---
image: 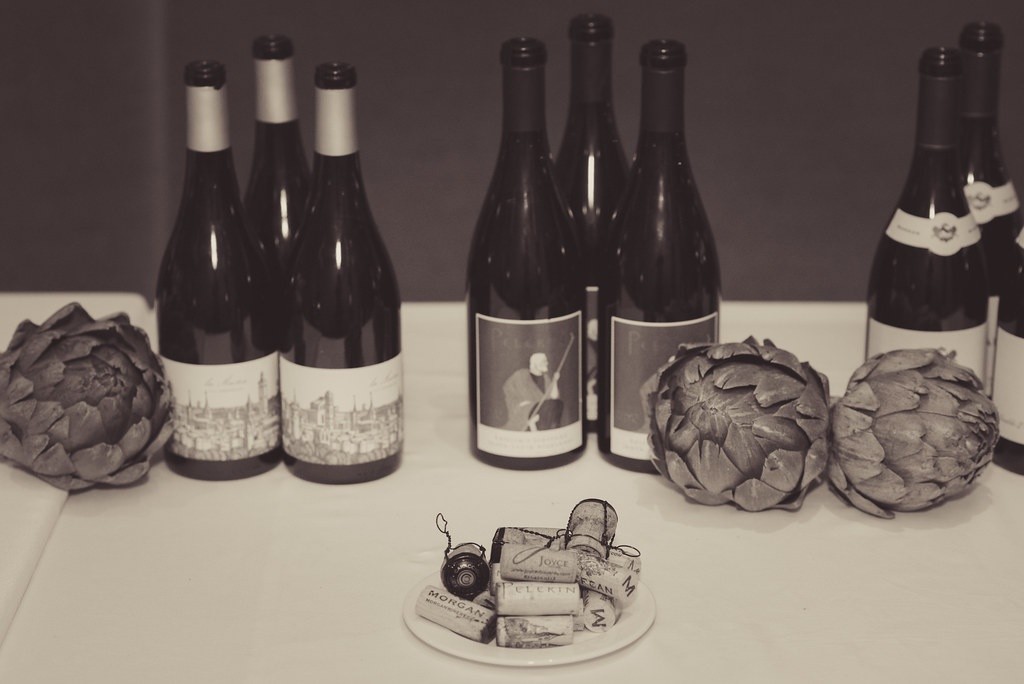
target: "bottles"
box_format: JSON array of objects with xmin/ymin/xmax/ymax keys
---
[
  {"xmin": 553, "ymin": 14, "xmax": 627, "ymax": 432},
  {"xmin": 468, "ymin": 37, "xmax": 586, "ymax": 470},
  {"xmin": 157, "ymin": 59, "xmax": 282, "ymax": 480},
  {"xmin": 279, "ymin": 62, "xmax": 404, "ymax": 484},
  {"xmin": 244, "ymin": 35, "xmax": 310, "ymax": 305},
  {"xmin": 866, "ymin": 45, "xmax": 989, "ymax": 389},
  {"xmin": 959, "ymin": 20, "xmax": 1021, "ymax": 397},
  {"xmin": 598, "ymin": 38, "xmax": 720, "ymax": 474},
  {"xmin": 993, "ymin": 228, "xmax": 1024, "ymax": 475}
]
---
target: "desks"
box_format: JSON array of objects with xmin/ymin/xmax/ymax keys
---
[{"xmin": 0, "ymin": 293, "xmax": 1024, "ymax": 684}]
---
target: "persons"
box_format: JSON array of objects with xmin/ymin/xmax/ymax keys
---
[{"xmin": 519, "ymin": 351, "xmax": 565, "ymax": 431}]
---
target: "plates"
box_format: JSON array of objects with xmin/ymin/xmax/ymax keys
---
[{"xmin": 403, "ymin": 569, "xmax": 655, "ymax": 667}]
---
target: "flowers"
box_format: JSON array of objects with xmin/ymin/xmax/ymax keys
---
[
  {"xmin": 825, "ymin": 350, "xmax": 1001, "ymax": 519},
  {"xmin": 639, "ymin": 337, "xmax": 831, "ymax": 512},
  {"xmin": 0, "ymin": 302, "xmax": 174, "ymax": 489}
]
